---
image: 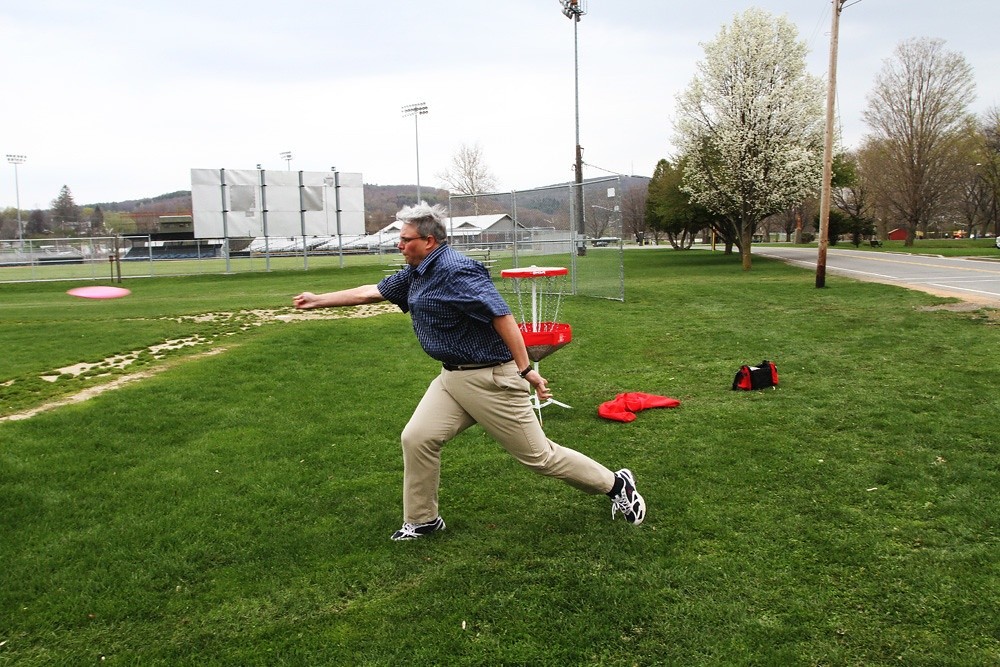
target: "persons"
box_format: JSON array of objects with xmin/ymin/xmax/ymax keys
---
[{"xmin": 294, "ymin": 204, "xmax": 648, "ymax": 541}]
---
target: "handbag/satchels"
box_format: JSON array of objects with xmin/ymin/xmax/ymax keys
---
[{"xmin": 732, "ymin": 360, "xmax": 779, "ymax": 390}]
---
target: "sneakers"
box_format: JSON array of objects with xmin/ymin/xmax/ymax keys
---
[
  {"xmin": 390, "ymin": 516, "xmax": 446, "ymax": 541},
  {"xmin": 611, "ymin": 467, "xmax": 646, "ymax": 525}
]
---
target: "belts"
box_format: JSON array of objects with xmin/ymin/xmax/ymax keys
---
[{"xmin": 443, "ymin": 358, "xmax": 513, "ymax": 372}]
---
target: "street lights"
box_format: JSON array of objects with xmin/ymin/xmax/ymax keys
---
[
  {"xmin": 555, "ymin": 0, "xmax": 589, "ymax": 258},
  {"xmin": 399, "ymin": 101, "xmax": 429, "ymax": 207},
  {"xmin": 279, "ymin": 150, "xmax": 292, "ymax": 172},
  {"xmin": 5, "ymin": 154, "xmax": 27, "ymax": 249}
]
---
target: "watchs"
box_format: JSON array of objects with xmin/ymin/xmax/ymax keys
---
[{"xmin": 517, "ymin": 364, "xmax": 532, "ymax": 378}]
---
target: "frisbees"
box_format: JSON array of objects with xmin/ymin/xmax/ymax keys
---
[{"xmin": 67, "ymin": 286, "xmax": 131, "ymax": 299}]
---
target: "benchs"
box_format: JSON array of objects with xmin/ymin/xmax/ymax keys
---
[
  {"xmin": 465, "ymin": 250, "xmax": 490, "ymax": 261},
  {"xmin": 385, "ymin": 266, "xmax": 492, "ymax": 279},
  {"xmin": 870, "ymin": 240, "xmax": 883, "ymax": 248}
]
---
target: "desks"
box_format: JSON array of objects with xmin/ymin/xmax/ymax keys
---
[{"xmin": 389, "ymin": 259, "xmax": 497, "ymax": 265}]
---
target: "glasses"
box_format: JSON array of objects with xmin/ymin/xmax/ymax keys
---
[{"xmin": 399, "ymin": 235, "xmax": 428, "ymax": 243}]
